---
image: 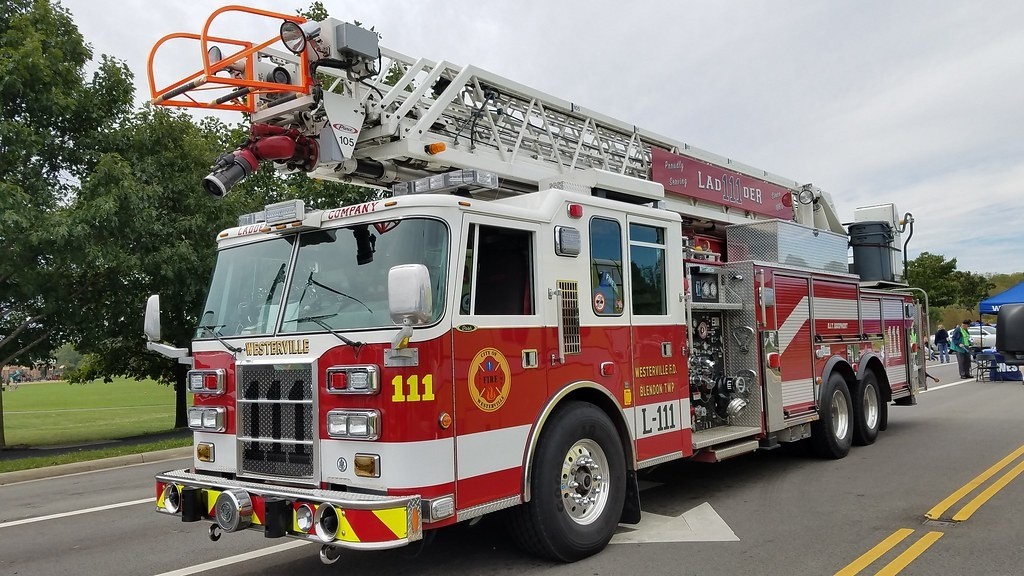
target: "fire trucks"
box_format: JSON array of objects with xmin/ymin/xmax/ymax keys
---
[{"xmin": 144, "ymin": 5, "xmax": 939, "ymax": 560}]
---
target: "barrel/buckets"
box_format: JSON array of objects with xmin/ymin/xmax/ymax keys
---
[
  {"xmin": 850, "ymin": 233, "xmax": 893, "ymax": 282},
  {"xmin": 849, "ymin": 221, "xmax": 890, "ymax": 235}
]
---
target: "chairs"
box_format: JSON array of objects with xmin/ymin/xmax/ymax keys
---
[{"xmin": 968, "ymin": 347, "xmax": 1004, "ymax": 383}]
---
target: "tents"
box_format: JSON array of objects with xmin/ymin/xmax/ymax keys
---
[{"xmin": 978, "ymin": 281, "xmax": 1024, "ymax": 377}]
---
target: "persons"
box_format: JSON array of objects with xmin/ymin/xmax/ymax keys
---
[
  {"xmin": 950, "ymin": 321, "xmax": 974, "ymax": 379},
  {"xmin": 934, "ymin": 325, "xmax": 950, "ymax": 363},
  {"xmin": 923, "ymin": 337, "xmax": 938, "ymax": 362}
]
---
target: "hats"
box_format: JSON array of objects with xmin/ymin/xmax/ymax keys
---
[{"xmin": 962, "ymin": 320, "xmax": 969, "ymax": 324}]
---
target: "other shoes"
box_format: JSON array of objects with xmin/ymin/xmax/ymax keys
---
[
  {"xmin": 961, "ymin": 375, "xmax": 966, "ymax": 379},
  {"xmin": 966, "ymin": 374, "xmax": 974, "ymax": 378}
]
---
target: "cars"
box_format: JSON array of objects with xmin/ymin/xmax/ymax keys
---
[
  {"xmin": 928, "ymin": 327, "xmax": 996, "ymax": 350},
  {"xmin": 975, "ymin": 324, "xmax": 996, "ymax": 334}
]
